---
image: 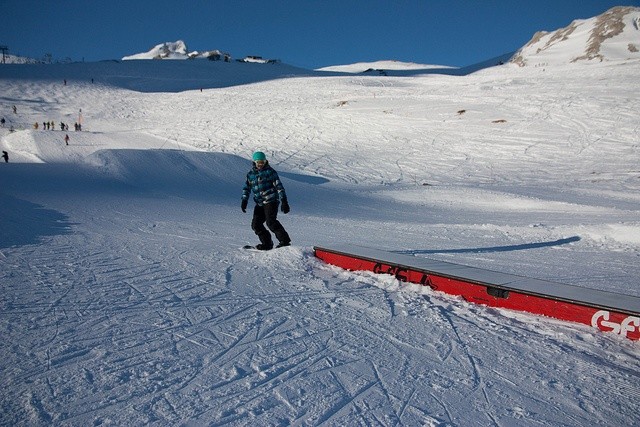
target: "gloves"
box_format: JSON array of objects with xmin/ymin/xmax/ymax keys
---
[
  {"xmin": 281, "ymin": 197, "xmax": 290, "ymax": 213},
  {"xmin": 241, "ymin": 199, "xmax": 248, "ymax": 213}
]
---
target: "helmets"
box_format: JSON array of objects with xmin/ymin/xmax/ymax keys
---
[{"xmin": 252, "ymin": 151, "xmax": 266, "ymax": 162}]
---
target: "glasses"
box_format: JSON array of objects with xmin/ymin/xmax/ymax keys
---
[{"xmin": 255, "ymin": 160, "xmax": 266, "ymax": 165}]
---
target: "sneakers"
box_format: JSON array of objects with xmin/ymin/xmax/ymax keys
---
[
  {"xmin": 256, "ymin": 243, "xmax": 273, "ymax": 250},
  {"xmin": 277, "ymin": 241, "xmax": 290, "ymax": 248}
]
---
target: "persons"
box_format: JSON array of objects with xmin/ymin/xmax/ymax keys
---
[
  {"xmin": 59, "ymin": 122, "xmax": 66, "ymax": 131},
  {"xmin": 74, "ymin": 122, "xmax": 82, "ymax": 131},
  {"xmin": 2, "ymin": 150, "xmax": 9, "ymax": 163},
  {"xmin": 33, "ymin": 121, "xmax": 38, "ymax": 128},
  {"xmin": 43, "ymin": 122, "xmax": 46, "ymax": 130},
  {"xmin": 241, "ymin": 151, "xmax": 291, "ymax": 250},
  {"xmin": 51, "ymin": 121, "xmax": 55, "ymax": 130},
  {"xmin": 64, "ymin": 134, "xmax": 69, "ymax": 145},
  {"xmin": 66, "ymin": 124, "xmax": 69, "ymax": 131},
  {"xmin": 46, "ymin": 121, "xmax": 51, "ymax": 130}
]
